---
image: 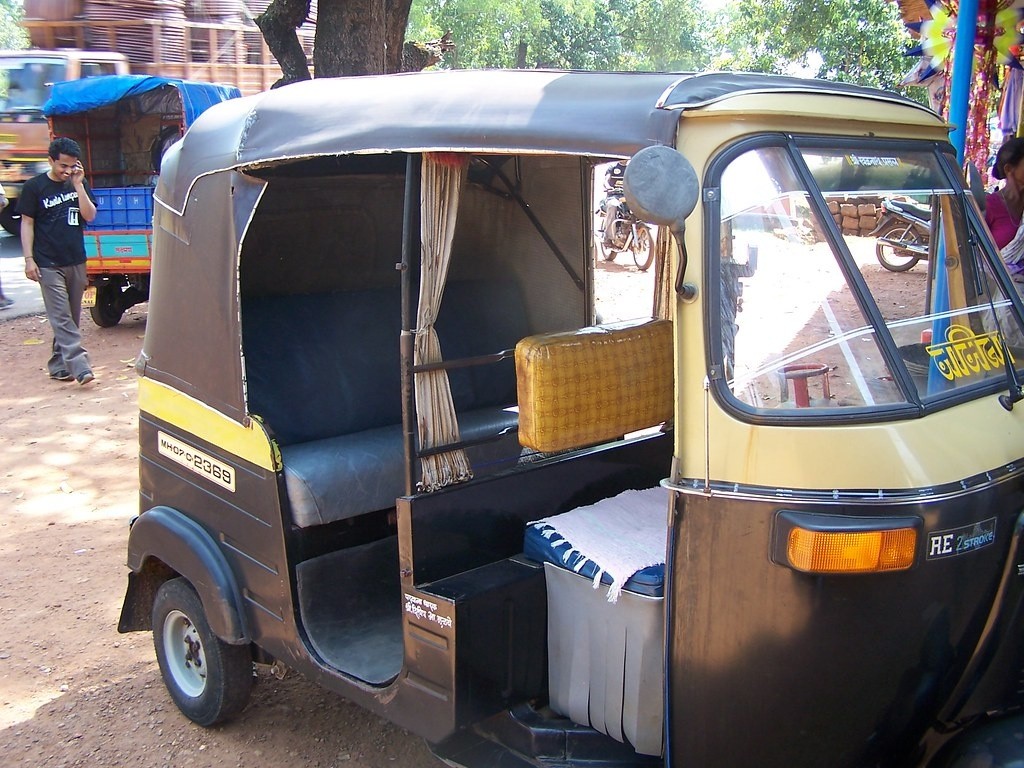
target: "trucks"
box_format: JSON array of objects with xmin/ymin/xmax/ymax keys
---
[{"xmin": 0, "ymin": 20, "xmax": 315, "ymax": 237}]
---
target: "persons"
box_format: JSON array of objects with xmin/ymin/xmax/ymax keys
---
[
  {"xmin": 0, "ymin": 182, "xmax": 16, "ymax": 310},
  {"xmin": 980, "ymin": 137, "xmax": 1024, "ymax": 348},
  {"xmin": 14, "ymin": 138, "xmax": 100, "ymax": 386},
  {"xmin": 603, "ymin": 160, "xmax": 628, "ymax": 246}
]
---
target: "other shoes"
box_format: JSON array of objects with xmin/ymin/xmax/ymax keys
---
[{"xmin": 604, "ymin": 237, "xmax": 612, "ymax": 244}]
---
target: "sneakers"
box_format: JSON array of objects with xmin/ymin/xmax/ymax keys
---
[
  {"xmin": 78, "ymin": 370, "xmax": 95, "ymax": 384},
  {"xmin": 0, "ymin": 297, "xmax": 16, "ymax": 310},
  {"xmin": 50, "ymin": 370, "xmax": 75, "ymax": 382}
]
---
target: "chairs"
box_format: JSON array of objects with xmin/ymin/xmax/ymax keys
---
[{"xmin": 516, "ymin": 314, "xmax": 674, "ymax": 755}]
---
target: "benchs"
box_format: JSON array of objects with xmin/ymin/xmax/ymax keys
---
[{"xmin": 244, "ymin": 273, "xmax": 530, "ymax": 562}]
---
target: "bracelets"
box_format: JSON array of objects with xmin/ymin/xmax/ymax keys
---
[{"xmin": 25, "ymin": 256, "xmax": 34, "ymax": 259}]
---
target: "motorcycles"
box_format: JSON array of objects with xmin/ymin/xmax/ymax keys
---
[
  {"xmin": 595, "ymin": 176, "xmax": 656, "ymax": 273},
  {"xmin": 867, "ymin": 194, "xmax": 932, "ymax": 273},
  {"xmin": 115, "ymin": 63, "xmax": 1022, "ymax": 767},
  {"xmin": 45, "ymin": 81, "xmax": 187, "ymax": 329}
]
